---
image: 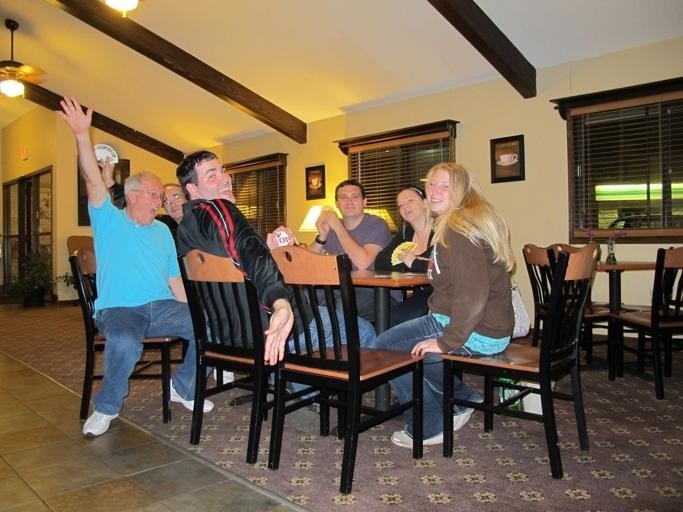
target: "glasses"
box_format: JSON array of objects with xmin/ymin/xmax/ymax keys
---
[{"xmin": 129, "ymin": 190, "xmax": 165, "ymax": 199}]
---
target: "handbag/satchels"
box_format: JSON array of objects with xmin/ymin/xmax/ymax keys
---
[{"xmin": 510, "ymin": 287, "xmax": 530, "ymax": 339}]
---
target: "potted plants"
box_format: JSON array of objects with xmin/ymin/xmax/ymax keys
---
[{"xmin": 5, "ymin": 242, "xmax": 77, "ymax": 307}]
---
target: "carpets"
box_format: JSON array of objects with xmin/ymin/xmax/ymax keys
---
[{"xmin": 0, "ymin": 308, "xmax": 681, "ymax": 512}]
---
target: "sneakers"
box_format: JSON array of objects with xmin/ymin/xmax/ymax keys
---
[
  {"xmin": 82, "ymin": 410, "xmax": 119, "ymax": 437},
  {"xmin": 391, "ymin": 430, "xmax": 443, "ymax": 448},
  {"xmin": 453, "ymin": 400, "xmax": 484, "ymax": 432},
  {"xmin": 170, "ymin": 378, "xmax": 215, "ymax": 412},
  {"xmin": 213, "ymin": 369, "xmax": 234, "ymax": 385}
]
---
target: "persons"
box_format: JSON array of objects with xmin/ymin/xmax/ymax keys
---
[
  {"xmin": 371, "ymin": 186, "xmax": 444, "ymax": 327},
  {"xmin": 96, "ymin": 158, "xmax": 189, "ymax": 234},
  {"xmin": 368, "ymin": 163, "xmax": 515, "ymax": 449},
  {"xmin": 175, "ymin": 150, "xmax": 376, "ymax": 400},
  {"xmin": 304, "ymin": 180, "xmax": 402, "ymax": 323},
  {"xmin": 55, "ymin": 96, "xmax": 214, "ymax": 439}
]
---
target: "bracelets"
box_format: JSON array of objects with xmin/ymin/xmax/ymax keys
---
[{"xmin": 315, "ymin": 235, "xmax": 327, "ymax": 245}]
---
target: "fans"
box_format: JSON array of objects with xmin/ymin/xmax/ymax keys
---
[{"xmin": 0, "ymin": 19, "xmax": 44, "ymax": 84}]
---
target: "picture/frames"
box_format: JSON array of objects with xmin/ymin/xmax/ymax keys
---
[
  {"xmin": 490, "ymin": 135, "xmax": 524, "ymax": 184},
  {"xmin": 305, "ymin": 165, "xmax": 325, "ymax": 201}
]
